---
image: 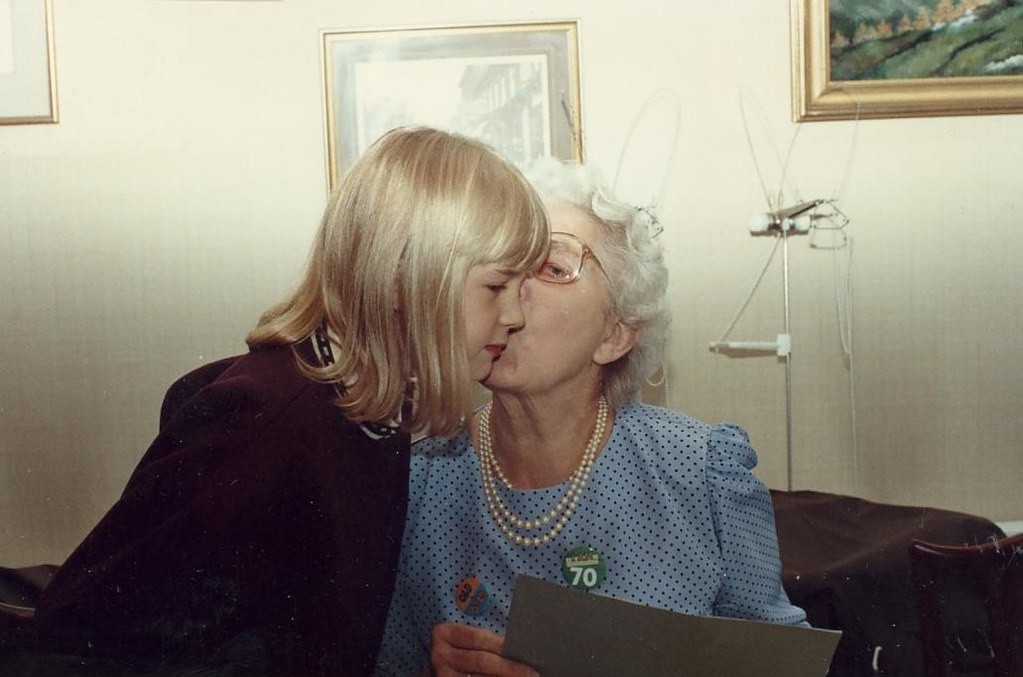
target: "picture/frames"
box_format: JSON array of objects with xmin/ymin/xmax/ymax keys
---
[
  {"xmin": 317, "ymin": 16, "xmax": 587, "ymax": 202},
  {"xmin": 787, "ymin": 0, "xmax": 1023, "ymax": 123},
  {"xmin": 0, "ymin": 0, "xmax": 58, "ymax": 125}
]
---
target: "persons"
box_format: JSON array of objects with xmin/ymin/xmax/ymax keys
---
[
  {"xmin": 376, "ymin": 159, "xmax": 811, "ymax": 677},
  {"xmin": 0, "ymin": 124, "xmax": 552, "ymax": 677}
]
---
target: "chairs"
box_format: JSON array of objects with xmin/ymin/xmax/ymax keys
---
[{"xmin": 906, "ymin": 533, "xmax": 1023, "ymax": 676}]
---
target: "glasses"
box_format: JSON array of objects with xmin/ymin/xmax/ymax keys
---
[{"xmin": 532, "ymin": 231, "xmax": 617, "ymax": 308}]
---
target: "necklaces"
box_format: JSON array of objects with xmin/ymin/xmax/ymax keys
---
[{"xmin": 479, "ymin": 395, "xmax": 607, "ymax": 545}]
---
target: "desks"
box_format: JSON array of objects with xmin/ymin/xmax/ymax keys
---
[{"xmin": 767, "ymin": 489, "xmax": 1023, "ymax": 677}]
